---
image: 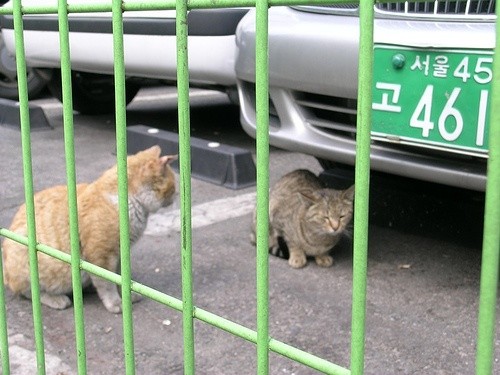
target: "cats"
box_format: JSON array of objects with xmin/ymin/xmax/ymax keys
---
[
  {"xmin": 250, "ymin": 168, "xmax": 355, "ymax": 269},
  {"xmin": 2, "ymin": 144, "xmax": 180, "ymax": 314}
]
